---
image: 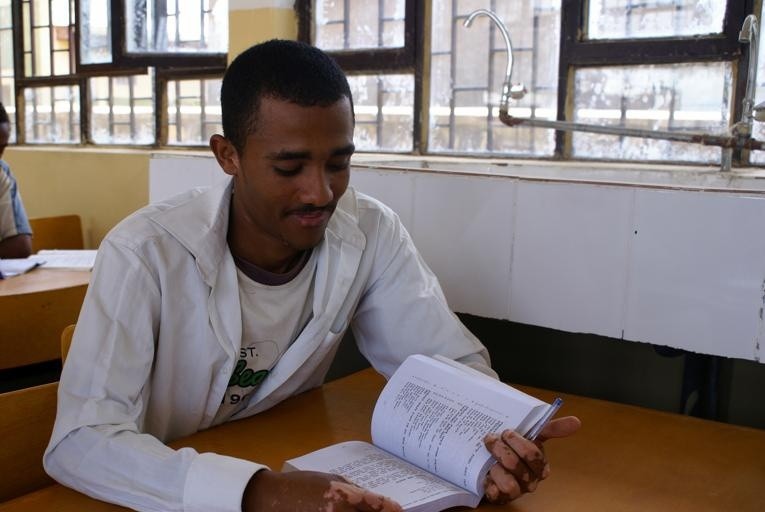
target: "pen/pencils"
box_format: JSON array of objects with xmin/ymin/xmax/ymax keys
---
[{"xmin": 525, "ymin": 397, "xmax": 564, "ymax": 442}]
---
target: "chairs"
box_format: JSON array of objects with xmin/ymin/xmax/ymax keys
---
[{"xmin": 29, "ymin": 215, "xmax": 87, "ymax": 256}]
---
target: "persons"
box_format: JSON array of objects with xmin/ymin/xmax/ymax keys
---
[
  {"xmin": 0, "ymin": 101, "xmax": 34, "ymax": 258},
  {"xmin": 43, "ymin": 39, "xmax": 582, "ymax": 512}
]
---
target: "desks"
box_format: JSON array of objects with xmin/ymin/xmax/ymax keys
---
[{"xmin": 1, "ymin": 258, "xmax": 765, "ymax": 511}]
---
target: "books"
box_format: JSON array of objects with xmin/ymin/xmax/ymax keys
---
[
  {"xmin": 0, "ymin": 259, "xmax": 48, "ymax": 279},
  {"xmin": 281, "ymin": 353, "xmax": 554, "ymax": 512},
  {"xmin": 28, "ymin": 249, "xmax": 99, "ymax": 272}
]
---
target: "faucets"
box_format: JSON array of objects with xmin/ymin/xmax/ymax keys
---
[
  {"xmin": 738, "ymin": 14, "xmax": 765, "ymax": 128},
  {"xmin": 463, "ymin": 8, "xmax": 526, "ymax": 115}
]
